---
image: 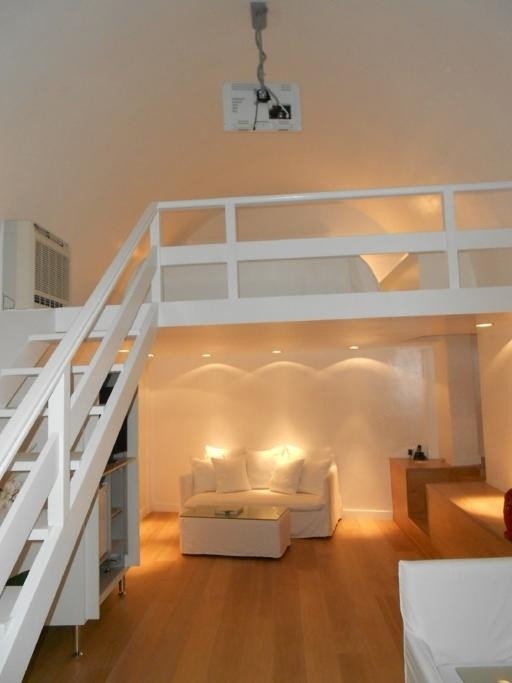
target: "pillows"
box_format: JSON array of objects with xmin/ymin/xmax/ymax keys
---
[{"xmin": 185, "ymin": 443, "xmax": 335, "ymax": 496}]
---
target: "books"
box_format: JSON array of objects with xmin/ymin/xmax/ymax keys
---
[{"xmin": 215, "ymin": 506, "xmax": 242, "ymax": 515}]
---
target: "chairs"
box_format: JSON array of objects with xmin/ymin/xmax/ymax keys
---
[{"xmin": 395, "ymin": 557, "xmax": 512, "ymax": 683}]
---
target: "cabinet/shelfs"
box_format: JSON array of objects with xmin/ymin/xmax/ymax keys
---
[{"xmin": 32, "ymin": 364, "xmax": 142, "ymax": 658}]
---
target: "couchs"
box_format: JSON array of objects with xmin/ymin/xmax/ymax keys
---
[{"xmin": 178, "ymin": 446, "xmax": 344, "ymax": 539}]
